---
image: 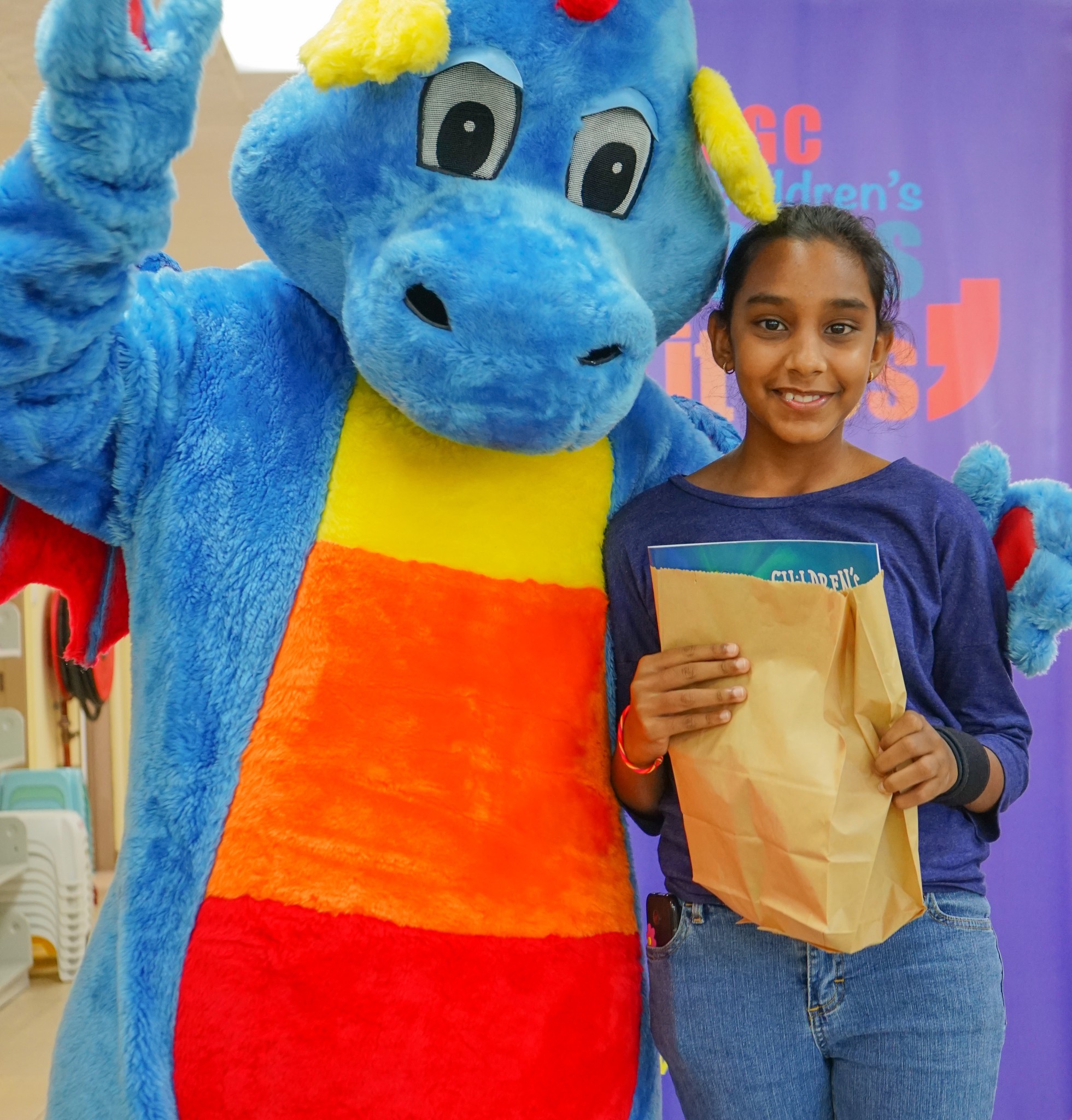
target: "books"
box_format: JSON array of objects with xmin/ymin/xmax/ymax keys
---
[{"xmin": 648, "ymin": 539, "xmax": 883, "ymax": 594}]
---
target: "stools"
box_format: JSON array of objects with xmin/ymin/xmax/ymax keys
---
[{"xmin": 0, "ymin": 765, "xmax": 96, "ymax": 983}]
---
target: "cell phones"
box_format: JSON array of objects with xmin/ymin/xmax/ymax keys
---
[{"xmin": 646, "ymin": 892, "xmax": 681, "ymax": 947}]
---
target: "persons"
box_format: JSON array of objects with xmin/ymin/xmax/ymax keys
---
[{"xmin": 595, "ymin": 201, "xmax": 1037, "ymax": 1120}]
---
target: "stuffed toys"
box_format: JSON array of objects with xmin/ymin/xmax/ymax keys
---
[{"xmin": 0, "ymin": 0, "xmax": 1072, "ymax": 1120}]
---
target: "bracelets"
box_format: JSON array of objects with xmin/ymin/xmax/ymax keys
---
[
  {"xmin": 618, "ymin": 704, "xmax": 663, "ymax": 774},
  {"xmin": 926, "ymin": 725, "xmax": 992, "ymax": 807}
]
